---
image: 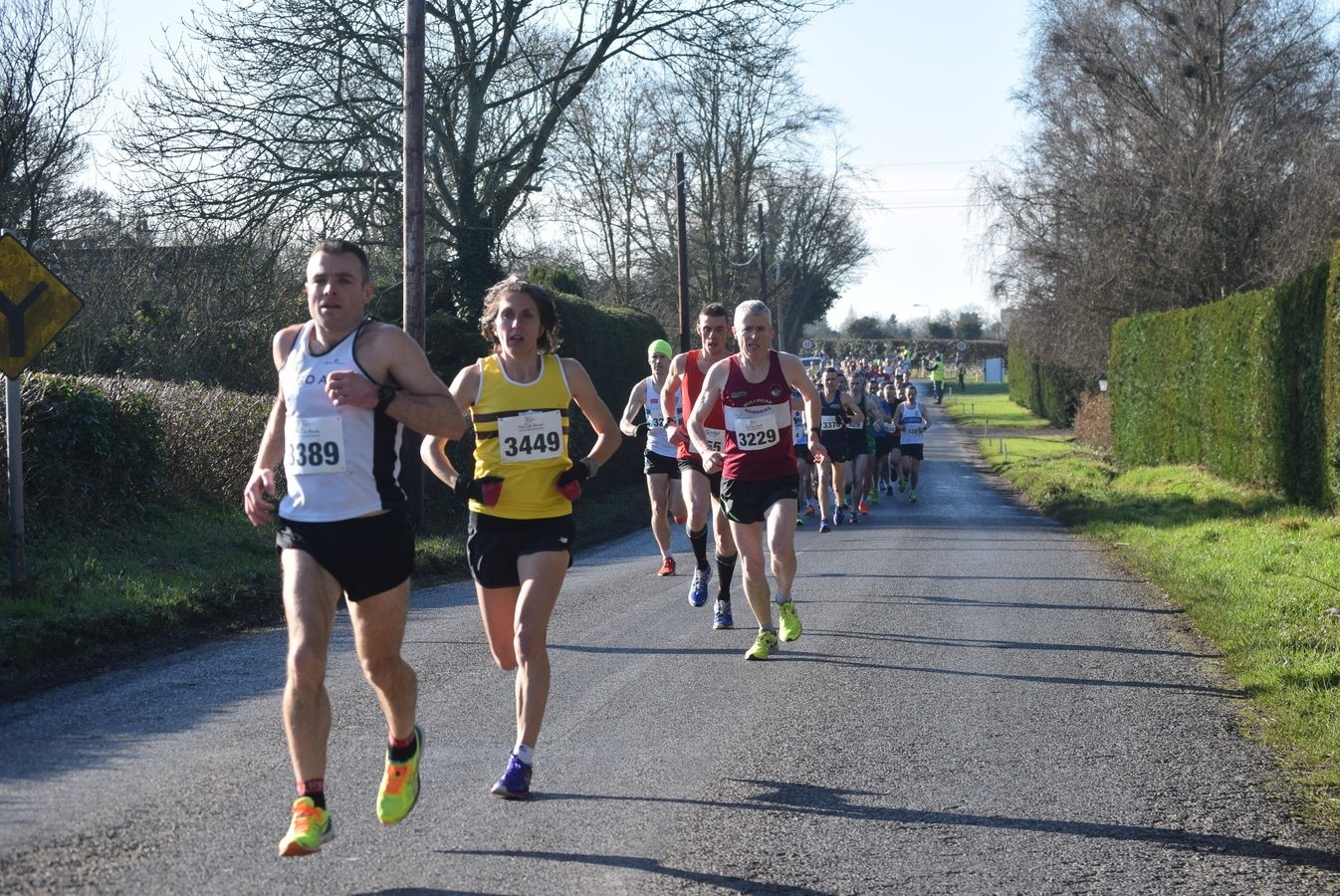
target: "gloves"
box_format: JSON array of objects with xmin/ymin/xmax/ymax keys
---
[
  {"xmin": 635, "ymin": 422, "xmax": 651, "ymax": 437},
  {"xmin": 453, "ymin": 474, "xmax": 504, "ymax": 507},
  {"xmin": 554, "ymin": 461, "xmax": 590, "ymax": 501}
]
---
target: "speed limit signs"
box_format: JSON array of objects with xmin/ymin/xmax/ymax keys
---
[
  {"xmin": 957, "ymin": 341, "xmax": 968, "ymax": 352},
  {"xmin": 802, "ymin": 340, "xmax": 812, "ymax": 351}
]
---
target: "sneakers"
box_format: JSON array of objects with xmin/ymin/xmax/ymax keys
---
[
  {"xmin": 279, "ymin": 796, "xmax": 336, "ymax": 855},
  {"xmin": 745, "ymin": 629, "xmax": 779, "ymax": 660},
  {"xmin": 775, "ymin": 595, "xmax": 803, "ymax": 642},
  {"xmin": 848, "ymin": 510, "xmax": 860, "ymax": 524},
  {"xmin": 812, "ymin": 468, "xmax": 917, "ymax": 503},
  {"xmin": 491, "ymin": 750, "xmax": 532, "ymax": 797},
  {"xmin": 805, "ymin": 505, "xmax": 814, "ymax": 515},
  {"xmin": 819, "ymin": 518, "xmax": 830, "ymax": 533},
  {"xmin": 688, "ymin": 564, "xmax": 713, "ymax": 607},
  {"xmin": 713, "ymin": 598, "xmax": 733, "ymax": 629},
  {"xmin": 674, "ymin": 515, "xmax": 686, "ymax": 524},
  {"xmin": 376, "ymin": 725, "xmax": 425, "ymax": 825},
  {"xmin": 796, "ymin": 517, "xmax": 804, "ymax": 526},
  {"xmin": 658, "ymin": 556, "xmax": 676, "ymax": 576},
  {"xmin": 859, "ymin": 501, "xmax": 868, "ymax": 515},
  {"xmin": 833, "ymin": 503, "xmax": 845, "ymax": 526}
]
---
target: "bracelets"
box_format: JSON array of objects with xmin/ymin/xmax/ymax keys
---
[
  {"xmin": 579, "ymin": 456, "xmax": 597, "ymax": 477},
  {"xmin": 923, "ymin": 426, "xmax": 926, "ymax": 430}
]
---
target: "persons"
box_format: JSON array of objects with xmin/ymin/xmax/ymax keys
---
[
  {"xmin": 686, "ymin": 299, "xmax": 829, "ymax": 661},
  {"xmin": 618, "ymin": 339, "xmax": 688, "ymax": 579},
  {"xmin": 790, "ymin": 347, "xmax": 944, "ymax": 534},
  {"xmin": 419, "ymin": 274, "xmax": 623, "ymax": 802},
  {"xmin": 660, "ymin": 303, "xmax": 738, "ymax": 629},
  {"xmin": 956, "ymin": 357, "xmax": 967, "ymax": 392},
  {"xmin": 244, "ymin": 238, "xmax": 465, "ymax": 857}
]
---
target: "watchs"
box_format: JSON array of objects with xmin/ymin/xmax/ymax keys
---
[
  {"xmin": 662, "ymin": 417, "xmax": 676, "ymax": 429},
  {"xmin": 811, "ymin": 427, "xmax": 821, "ymax": 438},
  {"xmin": 372, "ymin": 383, "xmax": 395, "ymax": 414}
]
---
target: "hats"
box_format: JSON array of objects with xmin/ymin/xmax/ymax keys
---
[{"xmin": 648, "ymin": 339, "xmax": 672, "ymax": 361}]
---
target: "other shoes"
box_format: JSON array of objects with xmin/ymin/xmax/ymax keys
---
[{"xmin": 844, "ymin": 502, "xmax": 849, "ymax": 509}]
